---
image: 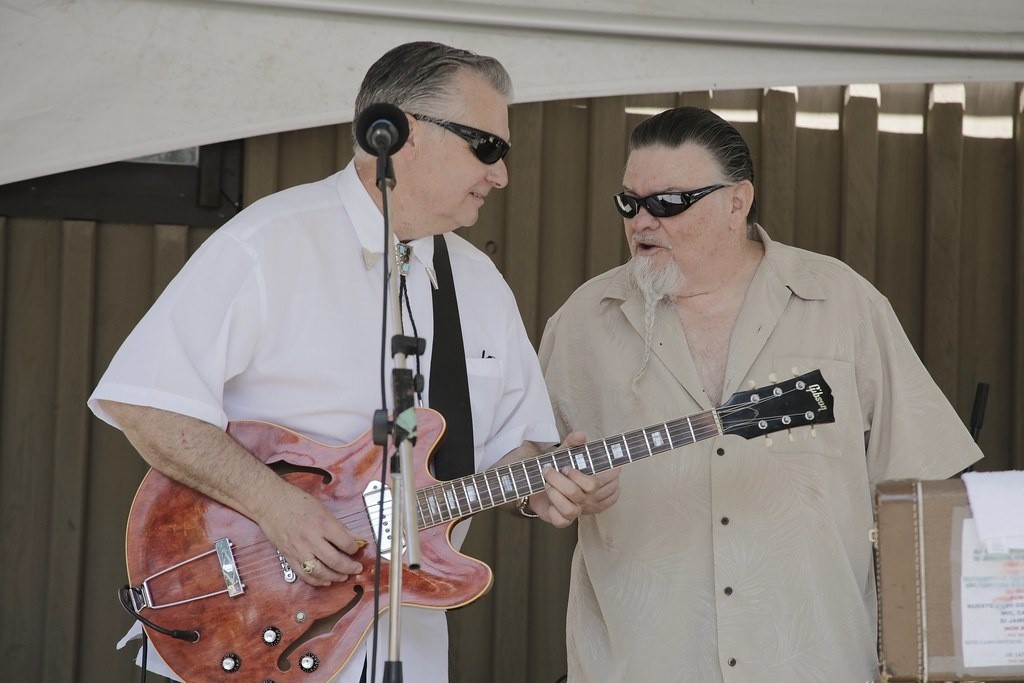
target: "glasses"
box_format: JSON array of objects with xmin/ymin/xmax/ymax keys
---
[
  {"xmin": 614, "ymin": 183, "xmax": 731, "ymax": 219},
  {"xmin": 406, "ymin": 112, "xmax": 510, "ymax": 164}
]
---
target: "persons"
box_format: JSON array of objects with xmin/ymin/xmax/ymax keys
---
[
  {"xmin": 535, "ymin": 106, "xmax": 986, "ymax": 683},
  {"xmin": 89, "ymin": 41, "xmax": 595, "ymax": 683}
]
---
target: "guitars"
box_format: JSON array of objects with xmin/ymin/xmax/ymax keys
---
[{"xmin": 119, "ymin": 358, "xmax": 839, "ymax": 682}]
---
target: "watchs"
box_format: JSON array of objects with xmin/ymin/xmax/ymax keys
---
[{"xmin": 516, "ymin": 497, "xmax": 539, "ymax": 518}]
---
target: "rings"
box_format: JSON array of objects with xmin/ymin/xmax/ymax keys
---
[{"xmin": 301, "ymin": 559, "xmax": 316, "ymax": 572}]
